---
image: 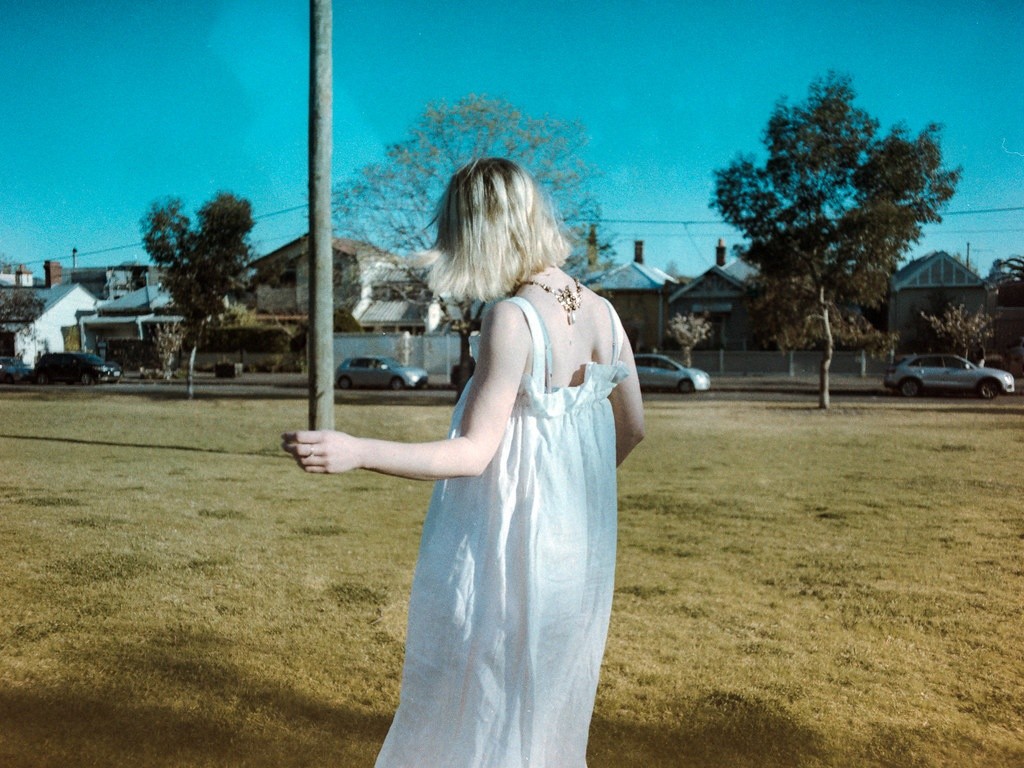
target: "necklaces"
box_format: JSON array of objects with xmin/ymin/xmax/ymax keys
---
[{"xmin": 512, "ymin": 277, "xmax": 583, "ymax": 324}]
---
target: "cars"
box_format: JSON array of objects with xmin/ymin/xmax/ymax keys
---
[{"xmin": 633, "ymin": 354, "xmax": 711, "ymax": 393}]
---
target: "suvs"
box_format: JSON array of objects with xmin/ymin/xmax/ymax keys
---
[
  {"xmin": 0, "ymin": 357, "xmax": 35, "ymax": 385},
  {"xmin": 334, "ymin": 354, "xmax": 429, "ymax": 391},
  {"xmin": 33, "ymin": 351, "xmax": 123, "ymax": 385},
  {"xmin": 882, "ymin": 352, "xmax": 1016, "ymax": 400}
]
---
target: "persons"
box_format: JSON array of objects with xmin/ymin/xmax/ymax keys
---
[{"xmin": 282, "ymin": 156, "xmax": 648, "ymax": 768}]
